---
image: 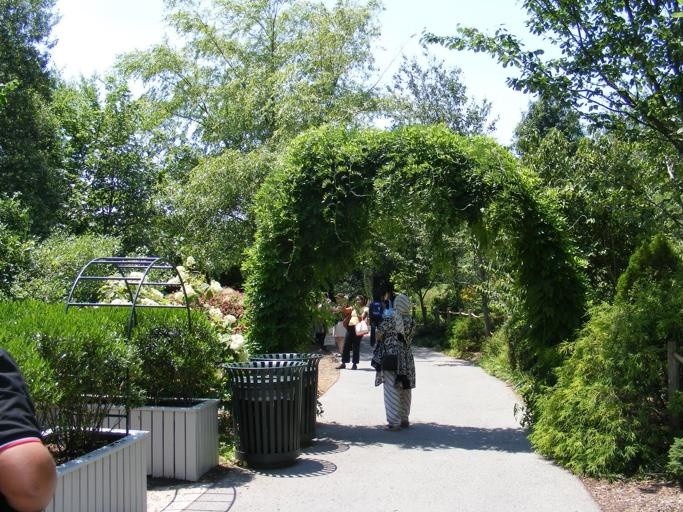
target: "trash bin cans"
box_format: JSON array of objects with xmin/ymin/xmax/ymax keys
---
[
  {"xmin": 248, "ymin": 350, "xmax": 325, "ymax": 446},
  {"xmin": 221, "ymin": 359, "xmax": 310, "ymax": 469}
]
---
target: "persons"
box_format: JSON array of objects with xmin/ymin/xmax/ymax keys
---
[
  {"xmin": 314, "ymin": 297, "xmax": 331, "ymax": 354},
  {"xmin": 333, "ymin": 294, "xmax": 371, "ymax": 370},
  {"xmin": 328, "ymin": 292, "xmax": 349, "ymax": 357},
  {"xmin": 0, "ymin": 347, "xmax": 58, "ymax": 511},
  {"xmin": 368, "ymin": 289, "xmax": 384, "ymax": 347},
  {"xmin": 371, "ymin": 294, "xmax": 415, "ymax": 431}
]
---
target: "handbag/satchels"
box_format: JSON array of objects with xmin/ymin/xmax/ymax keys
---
[{"xmin": 355, "ymin": 320, "xmax": 368, "ymax": 336}]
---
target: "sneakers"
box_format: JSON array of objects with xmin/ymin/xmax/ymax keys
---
[
  {"xmin": 350, "ymin": 365, "xmax": 357, "ymax": 369},
  {"xmin": 383, "ymin": 422, "xmax": 409, "ymax": 432},
  {"xmin": 335, "ymin": 363, "xmax": 346, "ymax": 369}
]
